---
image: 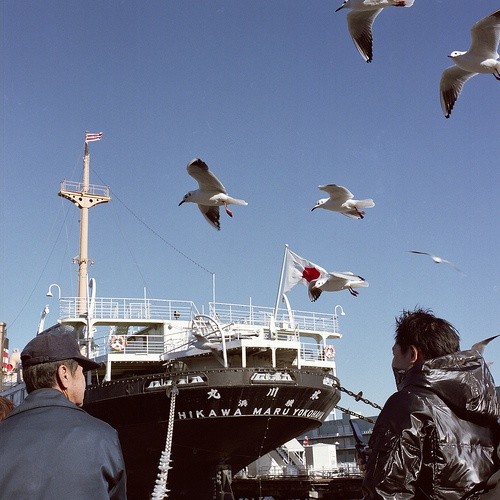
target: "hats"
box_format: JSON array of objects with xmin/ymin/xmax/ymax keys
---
[{"xmin": 20, "ymin": 331, "xmax": 100, "ymax": 372}]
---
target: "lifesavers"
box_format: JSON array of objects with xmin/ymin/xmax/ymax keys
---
[
  {"xmin": 324, "ymin": 346, "xmax": 336, "ymax": 358},
  {"xmin": 110, "ymin": 336, "xmax": 126, "ymax": 350}
]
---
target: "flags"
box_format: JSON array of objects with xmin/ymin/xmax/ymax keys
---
[
  {"xmin": 2, "ymin": 337, "xmax": 10, "ymax": 372},
  {"xmin": 86, "ymin": 132, "xmax": 103, "ymax": 142},
  {"xmin": 284, "ymin": 250, "xmax": 328, "ymax": 295}
]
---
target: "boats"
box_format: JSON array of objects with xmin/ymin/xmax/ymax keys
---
[{"xmin": 0, "ymin": 131, "xmax": 346, "ymax": 477}]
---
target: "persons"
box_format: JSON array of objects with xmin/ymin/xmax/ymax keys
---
[
  {"xmin": 354, "ymin": 305, "xmax": 500, "ymax": 500},
  {"xmin": 0, "ymin": 332, "xmax": 128, "ymax": 500}
]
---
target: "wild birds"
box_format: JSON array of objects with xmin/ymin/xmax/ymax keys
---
[
  {"xmin": 407, "ymin": 251, "xmax": 461, "ymax": 273},
  {"xmin": 309, "ymin": 272, "xmax": 369, "ymax": 302},
  {"xmin": 440, "ymin": 9, "xmax": 500, "ymax": 119},
  {"xmin": 336, "ymin": 0, "xmax": 415, "ymax": 63},
  {"xmin": 311, "ymin": 183, "xmax": 375, "ymax": 220},
  {"xmin": 179, "ymin": 158, "xmax": 249, "ymax": 231},
  {"xmin": 173, "ymin": 311, "xmax": 180, "ymax": 320},
  {"xmin": 470, "ymin": 334, "xmax": 500, "ymax": 366}
]
